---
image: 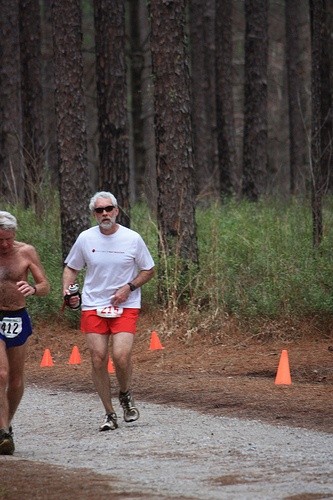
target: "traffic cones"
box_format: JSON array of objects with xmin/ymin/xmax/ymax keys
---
[
  {"xmin": 40, "ymin": 349, "xmax": 56, "ymax": 368},
  {"xmin": 274, "ymin": 350, "xmax": 293, "ymax": 386},
  {"xmin": 148, "ymin": 331, "xmax": 166, "ymax": 351},
  {"xmin": 67, "ymin": 345, "xmax": 81, "ymax": 366},
  {"xmin": 107, "ymin": 354, "xmax": 116, "ymax": 373}
]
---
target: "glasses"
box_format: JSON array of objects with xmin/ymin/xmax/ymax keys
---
[{"xmin": 94, "ymin": 206, "xmax": 116, "ymax": 213}]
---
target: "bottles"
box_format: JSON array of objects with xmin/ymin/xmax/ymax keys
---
[{"xmin": 69, "ymin": 283, "xmax": 83, "ymax": 312}]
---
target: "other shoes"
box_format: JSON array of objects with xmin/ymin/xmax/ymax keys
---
[{"xmin": 0, "ymin": 426, "xmax": 15, "ymax": 455}]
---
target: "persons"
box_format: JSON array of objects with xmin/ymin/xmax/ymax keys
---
[
  {"xmin": 0, "ymin": 210, "xmax": 50, "ymax": 454},
  {"xmin": 62, "ymin": 192, "xmax": 155, "ymax": 430}
]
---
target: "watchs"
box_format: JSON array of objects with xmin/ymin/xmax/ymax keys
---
[{"xmin": 127, "ymin": 283, "xmax": 135, "ymax": 291}]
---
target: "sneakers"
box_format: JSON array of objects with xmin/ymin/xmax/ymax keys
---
[
  {"xmin": 99, "ymin": 411, "xmax": 119, "ymax": 431},
  {"xmin": 119, "ymin": 390, "xmax": 140, "ymax": 423}
]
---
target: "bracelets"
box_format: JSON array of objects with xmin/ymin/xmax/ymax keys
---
[{"xmin": 30, "ymin": 285, "xmax": 36, "ymax": 295}]
